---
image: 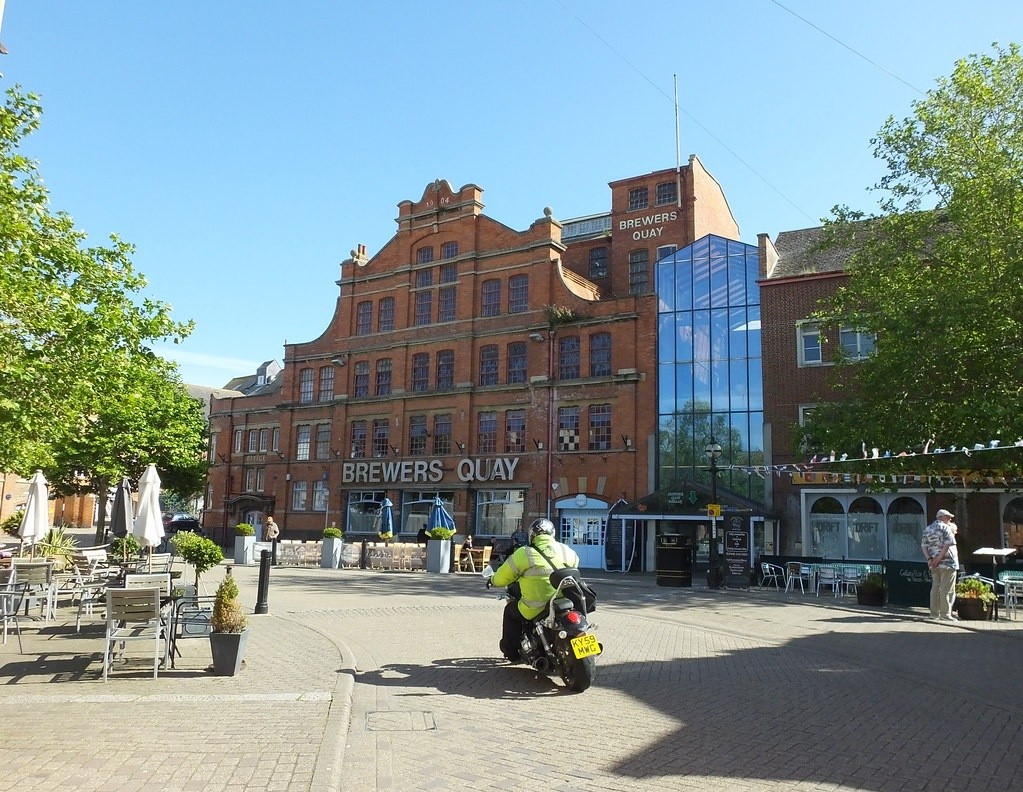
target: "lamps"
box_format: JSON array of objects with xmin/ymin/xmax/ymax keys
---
[
  {"xmin": 331, "ymin": 359, "xmax": 344, "ymax": 367},
  {"xmin": 530, "ymin": 334, "xmax": 545, "ymax": 343}
]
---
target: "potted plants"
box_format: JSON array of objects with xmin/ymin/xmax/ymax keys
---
[
  {"xmin": 206, "ymin": 574, "xmax": 248, "ymax": 676},
  {"xmin": 321, "ymin": 525, "xmax": 343, "ymax": 571},
  {"xmin": 856, "ymin": 572, "xmax": 888, "ymax": 606},
  {"xmin": 952, "ymin": 578, "xmax": 998, "ymax": 619},
  {"xmin": 235, "ymin": 519, "xmax": 257, "ymax": 565},
  {"xmin": 168, "ymin": 530, "xmax": 225, "ymax": 639},
  {"xmin": 425, "ymin": 526, "xmax": 458, "ymax": 573}
]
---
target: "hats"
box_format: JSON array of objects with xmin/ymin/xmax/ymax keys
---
[{"xmin": 936, "ymin": 509, "xmax": 954, "ymax": 518}]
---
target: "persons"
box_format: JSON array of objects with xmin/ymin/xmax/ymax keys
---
[
  {"xmin": 417, "ymin": 523, "xmax": 430, "ymax": 547},
  {"xmin": 488, "ymin": 536, "xmax": 500, "ymax": 560},
  {"xmin": 461, "ymin": 535, "xmax": 473, "ymax": 571},
  {"xmin": 485, "ymin": 518, "xmax": 579, "ymax": 662},
  {"xmin": 261, "ymin": 516, "xmax": 279, "ymax": 542},
  {"xmin": 921, "ymin": 510, "xmax": 958, "ymax": 621},
  {"xmin": 509, "ymin": 538, "xmax": 521, "ymax": 555}
]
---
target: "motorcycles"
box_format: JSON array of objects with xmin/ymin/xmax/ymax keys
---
[{"xmin": 481, "ymin": 567, "xmax": 603, "ymax": 693}]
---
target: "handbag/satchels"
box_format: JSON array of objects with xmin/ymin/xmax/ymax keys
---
[{"xmin": 562, "ymin": 586, "xmax": 596, "ymax": 615}]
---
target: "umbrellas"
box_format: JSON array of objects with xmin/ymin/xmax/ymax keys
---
[
  {"xmin": 17, "ymin": 469, "xmax": 50, "ymax": 615},
  {"xmin": 133, "ymin": 463, "xmax": 165, "ymax": 574},
  {"xmin": 378, "ymin": 497, "xmax": 393, "ymax": 547},
  {"xmin": 109, "ymin": 476, "xmax": 134, "ymax": 564},
  {"xmin": 425, "ymin": 493, "xmax": 456, "ymax": 539}
]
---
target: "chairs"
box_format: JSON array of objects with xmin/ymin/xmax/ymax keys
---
[
  {"xmin": 998, "ymin": 570, "xmax": 1023, "ymax": 621},
  {"xmin": 0, "ymin": 547, "xmax": 222, "ymax": 684},
  {"xmin": 801, "ymin": 562, "xmax": 813, "ymax": 591},
  {"xmin": 759, "ymin": 561, "xmax": 794, "ymax": 592},
  {"xmin": 785, "ymin": 561, "xmax": 806, "ymax": 595},
  {"xmin": 817, "ymin": 565, "xmax": 841, "ymax": 598},
  {"xmin": 264, "ymin": 533, "xmax": 493, "ymax": 577},
  {"xmin": 843, "ymin": 565, "xmax": 860, "ymax": 594}
]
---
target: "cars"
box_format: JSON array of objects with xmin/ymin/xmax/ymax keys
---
[{"xmin": 162, "ymin": 511, "xmax": 201, "ymax": 533}]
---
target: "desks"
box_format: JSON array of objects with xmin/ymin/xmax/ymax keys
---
[
  {"xmin": 112, "ymin": 593, "xmax": 184, "ymax": 667},
  {"xmin": 467, "ymin": 548, "xmax": 483, "ymax": 570},
  {"xmin": 117, "ymin": 569, "xmax": 184, "ymax": 588},
  {"xmin": 99, "ymin": 559, "xmax": 147, "ymax": 583}
]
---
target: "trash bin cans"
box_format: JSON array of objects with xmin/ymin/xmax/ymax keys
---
[{"xmin": 656, "ymin": 533, "xmax": 692, "ymax": 587}]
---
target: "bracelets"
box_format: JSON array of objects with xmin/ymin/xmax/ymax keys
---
[{"xmin": 928, "ymin": 557, "xmax": 933, "ymax": 561}]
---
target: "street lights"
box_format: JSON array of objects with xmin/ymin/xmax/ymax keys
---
[{"xmin": 703, "ymin": 436, "xmax": 726, "ymax": 590}]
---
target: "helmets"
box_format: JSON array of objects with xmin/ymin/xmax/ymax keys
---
[{"xmin": 528, "ymin": 518, "xmax": 555, "ymax": 546}]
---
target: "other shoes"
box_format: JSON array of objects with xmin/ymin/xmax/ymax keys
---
[
  {"xmin": 929, "ymin": 616, "xmax": 939, "ymax": 621},
  {"xmin": 500, "ymin": 639, "xmax": 520, "ymax": 661},
  {"xmin": 940, "ymin": 616, "xmax": 957, "ymax": 621}
]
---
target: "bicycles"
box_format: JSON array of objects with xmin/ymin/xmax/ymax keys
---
[{"xmin": 145, "ymin": 537, "xmax": 168, "ymax": 553}]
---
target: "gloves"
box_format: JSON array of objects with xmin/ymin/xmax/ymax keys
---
[{"xmin": 486, "ymin": 579, "xmax": 492, "ymax": 589}]
